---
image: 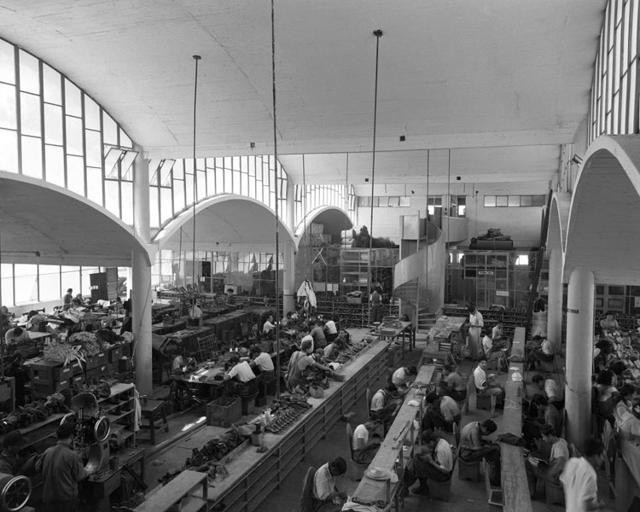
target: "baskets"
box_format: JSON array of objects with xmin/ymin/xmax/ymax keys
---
[{"xmin": 207, "ymin": 395, "xmax": 242, "ymax": 428}]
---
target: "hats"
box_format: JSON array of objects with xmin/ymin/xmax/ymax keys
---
[
  {"xmin": 57, "ymin": 423, "xmax": 74, "ymax": 439},
  {"xmin": 363, "ymin": 466, "xmax": 393, "ymax": 482}
]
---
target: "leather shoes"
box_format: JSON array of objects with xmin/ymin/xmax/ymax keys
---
[{"xmin": 402, "ymin": 486, "xmax": 430, "ymax": 496}]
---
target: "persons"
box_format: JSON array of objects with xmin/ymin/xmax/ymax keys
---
[
  {"xmin": 313, "ymin": 457, "xmax": 349, "ymax": 511},
  {"xmin": 371, "ymin": 385, "xmax": 402, "ymax": 422},
  {"xmin": 0, "ymin": 422, "xmax": 87, "ymax": 512},
  {"xmin": 170, "ymin": 285, "xmax": 382, "ymax": 396},
  {"xmin": 392, "ymin": 366, "xmax": 417, "ymax": 396},
  {"xmin": 593, "ymin": 331, "xmax": 640, "ymax": 437},
  {"xmin": 526, "ymin": 376, "xmax": 569, "ymax": 497},
  {"xmin": 600, "ymin": 312, "xmax": 618, "ymax": 328},
  {"xmin": 420, "ymin": 393, "xmax": 460, "ymax": 433},
  {"xmin": 440, "ymin": 365, "xmax": 467, "ymax": 401},
  {"xmin": 404, "ymin": 428, "xmax": 453, "ymax": 496},
  {"xmin": 351, "ymin": 413, "xmax": 382, "ymax": 464},
  {"xmin": 559, "ymin": 438, "xmax": 605, "ymax": 512},
  {"xmin": 466, "ymin": 303, "xmax": 504, "ymax": 369},
  {"xmin": 459, "ymin": 419, "xmax": 502, "ymax": 483},
  {"xmin": 473, "ymin": 358, "xmax": 506, "ymax": 411},
  {"xmin": 0, "ymin": 288, "xmax": 132, "ymax": 352},
  {"xmin": 527, "ymin": 300, "xmax": 553, "ymax": 370}
]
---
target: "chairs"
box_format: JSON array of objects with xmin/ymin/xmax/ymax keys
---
[{"xmin": 0, "ymin": 302, "xmax": 640, "ymax": 512}]
